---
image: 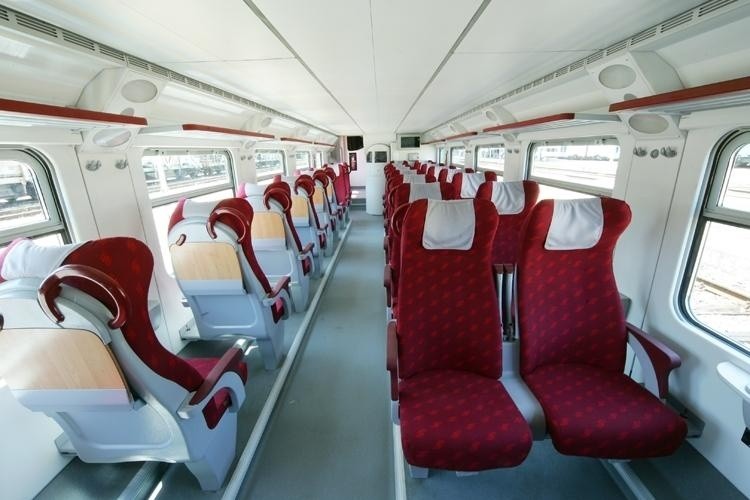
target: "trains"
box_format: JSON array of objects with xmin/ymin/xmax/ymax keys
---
[{"xmin": 0, "ymin": 151, "xmax": 307, "ymax": 204}]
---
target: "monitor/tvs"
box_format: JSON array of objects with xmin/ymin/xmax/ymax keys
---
[{"xmin": 398, "ymin": 134, "xmax": 421, "ymax": 150}]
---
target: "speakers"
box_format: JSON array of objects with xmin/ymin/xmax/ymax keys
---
[{"xmin": 347, "ymin": 136, "xmax": 364, "ymax": 151}]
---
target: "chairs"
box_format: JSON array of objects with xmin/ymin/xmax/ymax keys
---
[
  {"xmin": 381, "ymin": 158, "xmax": 703, "ymax": 480},
  {"xmin": 1, "ymin": 161, "xmax": 350, "ymax": 491}
]
